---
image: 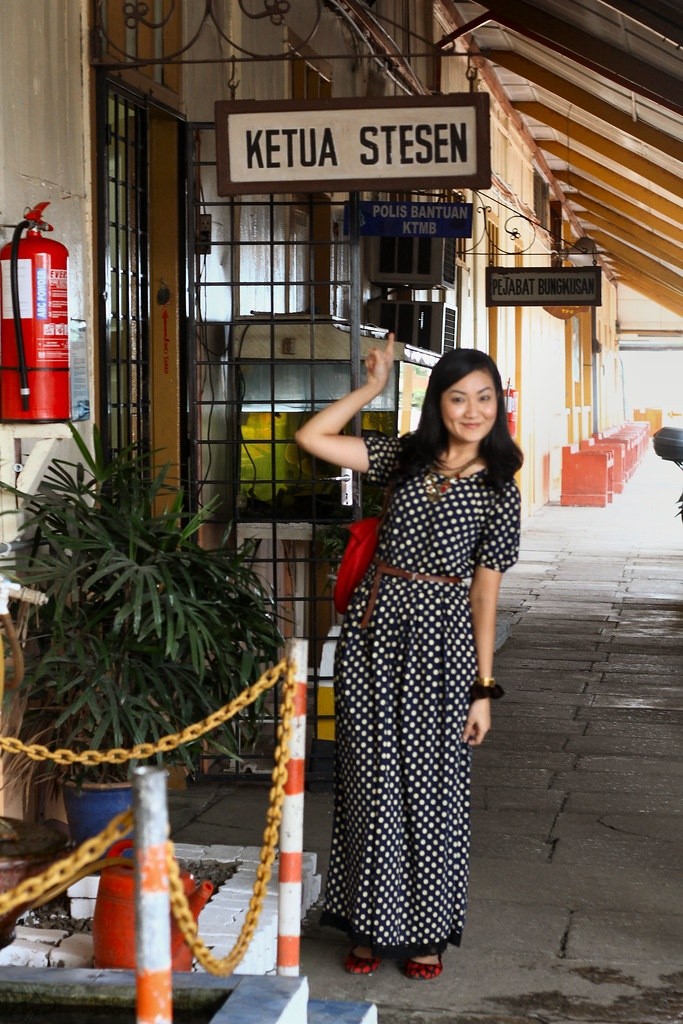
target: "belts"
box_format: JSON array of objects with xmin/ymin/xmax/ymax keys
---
[{"xmin": 359, "ymin": 547, "xmax": 463, "ymax": 629}]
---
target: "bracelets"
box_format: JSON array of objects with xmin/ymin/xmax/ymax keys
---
[{"xmin": 470, "ymin": 678, "xmax": 504, "ymax": 699}]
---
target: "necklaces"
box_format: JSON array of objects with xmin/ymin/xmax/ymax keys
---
[
  {"xmin": 425, "ymin": 456, "xmax": 480, "ymax": 502},
  {"xmin": 295, "ymin": 333, "xmax": 523, "ymax": 978}
]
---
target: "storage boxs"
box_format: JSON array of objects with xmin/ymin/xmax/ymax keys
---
[{"xmin": 226, "ymin": 316, "xmax": 441, "ymax": 529}]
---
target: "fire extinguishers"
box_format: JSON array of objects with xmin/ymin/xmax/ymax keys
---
[{"xmin": 0, "ymin": 199, "xmax": 74, "ymax": 427}]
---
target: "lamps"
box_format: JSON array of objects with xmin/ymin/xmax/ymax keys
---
[{"xmin": 551, "ymin": 238, "xmax": 597, "ymax": 268}]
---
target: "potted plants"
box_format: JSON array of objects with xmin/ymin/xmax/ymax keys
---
[{"xmin": 0, "ymin": 420, "xmax": 302, "ymax": 876}]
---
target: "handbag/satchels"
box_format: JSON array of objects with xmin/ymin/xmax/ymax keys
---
[{"xmin": 333, "ymin": 438, "xmax": 411, "ymax": 615}]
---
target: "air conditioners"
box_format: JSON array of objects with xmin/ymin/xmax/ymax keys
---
[
  {"xmin": 367, "ymin": 297, "xmax": 460, "ymax": 356},
  {"xmin": 366, "ymin": 238, "xmax": 458, "ymax": 289}
]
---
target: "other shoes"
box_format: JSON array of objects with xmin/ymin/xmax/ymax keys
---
[
  {"xmin": 404, "ymin": 953, "xmax": 444, "ymax": 981},
  {"xmin": 344, "ymin": 944, "xmax": 380, "ymax": 973}
]
